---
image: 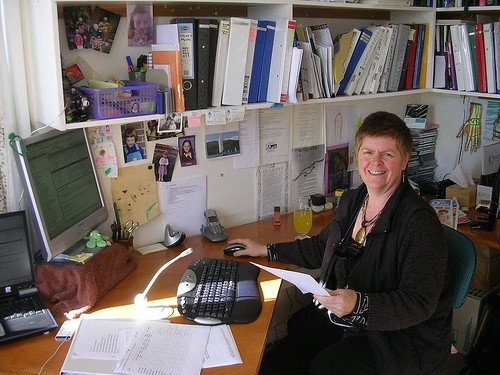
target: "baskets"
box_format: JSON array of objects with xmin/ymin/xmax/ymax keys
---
[{"xmin": 72, "ymin": 80, "xmax": 158, "ymax": 121}]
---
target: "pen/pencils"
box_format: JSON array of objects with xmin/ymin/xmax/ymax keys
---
[
  {"xmin": 113, "ymin": 202, "xmax": 121, "ymax": 239},
  {"xmin": 111, "ymin": 222, "xmax": 125, "ymax": 240}
]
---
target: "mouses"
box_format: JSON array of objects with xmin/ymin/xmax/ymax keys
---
[{"xmin": 223, "ymin": 243, "xmax": 247, "ymax": 256}]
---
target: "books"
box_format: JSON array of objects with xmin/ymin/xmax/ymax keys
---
[
  {"xmin": 434, "ymin": 15, "xmax": 500, "ymax": 94},
  {"xmin": 408, "ymin": 0, "xmax": 500, "ymax": 8},
  {"xmin": 430, "ymin": 199, "xmax": 470, "ymax": 230},
  {"xmin": 148, "ymin": 17, "xmax": 429, "ymax": 113}
]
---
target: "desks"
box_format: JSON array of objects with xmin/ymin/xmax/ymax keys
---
[{"xmin": 0, "ymin": 205, "xmax": 500, "ymax": 375}]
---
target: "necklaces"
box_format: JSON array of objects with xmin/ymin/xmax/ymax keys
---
[{"xmin": 354, "ymin": 191, "xmax": 382, "ymax": 245}]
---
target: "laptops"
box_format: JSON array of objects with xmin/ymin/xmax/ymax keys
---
[{"xmin": 0, "ymin": 209, "xmax": 59, "ymax": 345}]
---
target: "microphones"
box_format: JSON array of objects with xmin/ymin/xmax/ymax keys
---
[{"xmin": 134, "ymin": 247, "xmax": 194, "ymax": 305}]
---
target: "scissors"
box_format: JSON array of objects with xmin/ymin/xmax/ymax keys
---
[{"xmin": 125, "ymin": 219, "xmax": 139, "ymax": 239}]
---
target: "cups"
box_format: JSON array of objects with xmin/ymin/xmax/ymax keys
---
[{"xmin": 294, "ymin": 196, "xmax": 312, "ymax": 242}]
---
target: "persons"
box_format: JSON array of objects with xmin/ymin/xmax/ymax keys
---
[
  {"xmin": 128, "ymin": 5, "xmax": 153, "ymax": 47},
  {"xmin": 437, "ymin": 210, "xmax": 449, "ymax": 221},
  {"xmin": 227, "ymin": 110, "xmax": 452, "ymax": 375},
  {"xmin": 69, "ymin": 17, "xmax": 111, "ymax": 50},
  {"xmin": 158, "ymin": 152, "xmax": 169, "ymax": 181},
  {"xmin": 147, "ymin": 124, "xmax": 160, "ymax": 140},
  {"xmin": 182, "ymin": 140, "xmax": 194, "ymax": 162},
  {"xmin": 131, "ymin": 102, "xmax": 139, "ymax": 113},
  {"xmin": 328, "ymin": 152, "xmax": 347, "ymax": 192},
  {"xmin": 160, "ymin": 116, "xmax": 176, "ymax": 130},
  {"xmin": 123, "ymin": 127, "xmax": 146, "ymax": 163}
]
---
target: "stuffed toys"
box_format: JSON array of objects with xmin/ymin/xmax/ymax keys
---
[{"xmin": 83, "ymin": 230, "xmax": 109, "ymax": 248}]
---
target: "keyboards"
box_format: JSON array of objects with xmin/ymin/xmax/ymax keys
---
[{"xmin": 176, "ymin": 257, "xmax": 261, "ymax": 326}]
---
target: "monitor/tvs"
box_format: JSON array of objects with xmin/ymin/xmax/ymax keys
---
[{"xmin": 11, "ymin": 125, "xmax": 108, "ymax": 263}]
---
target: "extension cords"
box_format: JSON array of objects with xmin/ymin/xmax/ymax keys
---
[{"xmin": 310, "ymin": 202, "xmax": 334, "ymax": 212}]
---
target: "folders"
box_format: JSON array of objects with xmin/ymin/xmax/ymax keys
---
[
  {"xmin": 170, "ymin": 18, "xmax": 197, "ymax": 110},
  {"xmin": 257, "ymin": 21, "xmax": 276, "ymax": 102},
  {"xmin": 450, "ymin": 20, "xmax": 500, "ymax": 94},
  {"xmin": 337, "ymin": 27, "xmax": 372, "ymax": 94},
  {"xmin": 247, "ymin": 20, "xmax": 268, "ymax": 104},
  {"xmin": 195, "ymin": 18, "xmax": 210, "ymax": 110},
  {"xmin": 207, "ymin": 18, "xmax": 218, "ymax": 107}
]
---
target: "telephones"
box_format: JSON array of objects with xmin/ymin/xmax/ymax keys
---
[{"xmin": 200, "ymin": 209, "xmax": 229, "ymax": 242}]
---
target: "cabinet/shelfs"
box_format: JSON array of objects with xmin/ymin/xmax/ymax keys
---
[{"xmin": 23, "ymin": 0, "xmax": 500, "ymax": 131}]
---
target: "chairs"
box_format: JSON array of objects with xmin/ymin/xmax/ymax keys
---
[{"xmin": 439, "ymin": 222, "xmax": 480, "ymax": 308}]
